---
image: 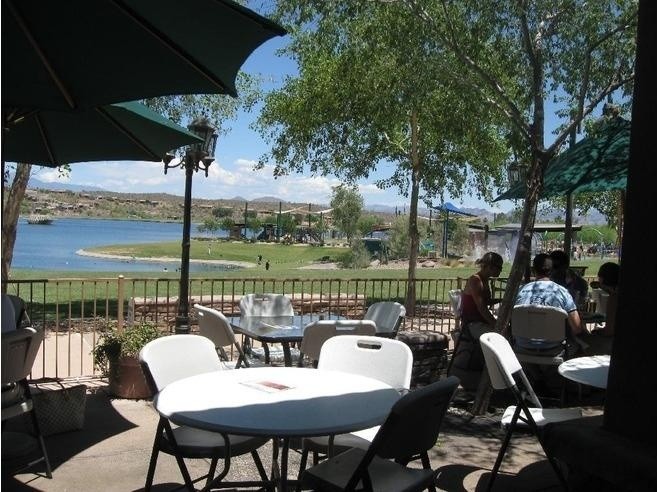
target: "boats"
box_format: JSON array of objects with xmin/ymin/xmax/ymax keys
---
[{"xmin": 24, "ymin": 214, "xmax": 53, "ymax": 225}]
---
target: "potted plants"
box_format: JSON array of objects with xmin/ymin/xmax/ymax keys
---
[{"xmin": 90, "ymin": 317, "xmax": 164, "ymax": 399}]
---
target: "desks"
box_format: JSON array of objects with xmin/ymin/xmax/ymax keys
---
[{"xmin": 155, "ymin": 366, "xmax": 401, "ymax": 490}]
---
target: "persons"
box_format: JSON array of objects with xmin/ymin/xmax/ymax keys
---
[
  {"xmin": 568, "ymin": 262, "xmax": 619, "ymax": 358},
  {"xmin": 2, "ymin": 258, "xmax": 27, "ymax": 406},
  {"xmin": 265, "ymin": 259, "xmax": 271, "ymax": 269},
  {"xmin": 461, "ymin": 251, "xmax": 511, "ymax": 345},
  {"xmin": 556, "ymin": 238, "xmax": 615, "ymax": 261},
  {"xmin": 552, "ymin": 250, "xmax": 587, "ymax": 303},
  {"xmin": 510, "ymin": 253, "xmax": 583, "ymax": 408},
  {"xmin": 257, "ymin": 253, "xmax": 262, "ymax": 265}
]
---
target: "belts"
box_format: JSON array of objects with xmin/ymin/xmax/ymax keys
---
[{"xmin": 526, "ymin": 346, "xmax": 559, "ymax": 353}]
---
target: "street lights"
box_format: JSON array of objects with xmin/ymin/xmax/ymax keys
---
[
  {"xmin": 162, "ymin": 105, "xmax": 219, "ymax": 334},
  {"xmin": 507, "ymin": 153, "xmax": 530, "ymax": 285}
]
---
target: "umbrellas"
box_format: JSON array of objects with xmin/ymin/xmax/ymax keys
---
[
  {"xmin": 491, "ymin": 113, "xmax": 630, "ymax": 203},
  {"xmin": 1, "ymin": 99, "xmax": 205, "ymax": 228},
  {"xmin": 2, "ymin": 0, "xmax": 288, "ymax": 116}
]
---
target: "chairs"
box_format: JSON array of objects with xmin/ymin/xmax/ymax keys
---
[
  {"xmin": 7, "ymin": 294, "xmax": 27, "ymax": 328},
  {"xmin": 194, "ymin": 294, "xmax": 407, "ymax": 369},
  {"xmin": 137, "ymin": 334, "xmax": 275, "ymax": 491},
  {"xmin": 304, "ymin": 378, "xmax": 458, "ymax": 490},
  {"xmin": 447, "ymin": 285, "xmax": 610, "ymax": 492},
  {"xmin": 1, "ymin": 327, "xmax": 52, "ymax": 478},
  {"xmin": 282, "ymin": 334, "xmax": 413, "ymax": 476}
]
